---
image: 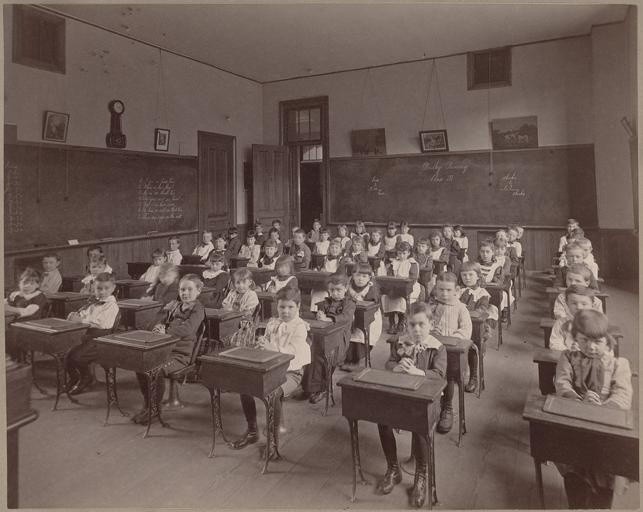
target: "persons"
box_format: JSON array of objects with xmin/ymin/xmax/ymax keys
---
[
  {"xmin": 85, "ymin": 247, "xmax": 104, "ymax": 275},
  {"xmin": 61, "ymin": 274, "xmax": 119, "ymax": 395},
  {"xmin": 458, "ymin": 261, "xmax": 492, "ymax": 393},
  {"xmin": 550, "ymin": 285, "xmax": 597, "ymax": 350},
  {"xmin": 4, "ymin": 269, "xmax": 49, "ymax": 361},
  {"xmin": 340, "ymin": 264, "xmax": 382, "ymax": 372},
  {"xmin": 39, "ymin": 253, "xmax": 63, "ymax": 292},
  {"xmin": 222, "ymin": 269, "xmax": 259, "ymax": 348},
  {"xmin": 140, "ymin": 248, "xmax": 168, "ymax": 294},
  {"xmin": 79, "ymin": 254, "xmax": 112, "ymax": 294},
  {"xmin": 433, "ymin": 272, "xmax": 473, "ymax": 433},
  {"xmin": 199, "ymin": 251, "xmax": 229, "ymax": 309},
  {"xmin": 131, "ymin": 274, "xmax": 204, "ymax": 424},
  {"xmin": 309, "ymin": 275, "xmax": 356, "ymax": 403},
  {"xmin": 557, "ymin": 309, "xmax": 631, "ymax": 511},
  {"xmin": 376, "ymin": 304, "xmax": 447, "ymax": 508},
  {"xmin": 195, "ymin": 219, "xmax": 526, "ymax": 323},
  {"xmin": 152, "ymin": 263, "xmax": 179, "ymax": 306},
  {"xmin": 230, "ymin": 288, "xmax": 311, "ymax": 459},
  {"xmin": 266, "ymin": 256, "xmax": 297, "ymax": 294},
  {"xmin": 166, "ymin": 236, "xmax": 183, "ymax": 265},
  {"xmin": 557, "ymin": 219, "xmax": 597, "ymax": 287}
]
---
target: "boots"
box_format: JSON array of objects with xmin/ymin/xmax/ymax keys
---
[
  {"xmin": 411, "ymin": 444, "xmax": 428, "ymax": 508},
  {"xmin": 301, "ymin": 390, "xmax": 318, "ymax": 400},
  {"xmin": 377, "ymin": 441, "xmax": 402, "ymax": 494},
  {"xmin": 234, "ymin": 398, "xmax": 260, "ymax": 449},
  {"xmin": 130, "ymin": 385, "xmax": 149, "ymax": 420},
  {"xmin": 135, "ymin": 386, "xmax": 164, "ymax": 423},
  {"xmin": 348, "ymin": 343, "xmax": 368, "ymax": 371},
  {"xmin": 309, "ymin": 390, "xmax": 327, "ymax": 404},
  {"xmin": 438, "ymin": 380, "xmax": 453, "ymax": 432},
  {"xmin": 397, "ymin": 312, "xmax": 404, "ymax": 332},
  {"xmin": 388, "ymin": 313, "xmax": 398, "ymax": 335},
  {"xmin": 465, "ymin": 351, "xmax": 477, "ymax": 393},
  {"xmin": 340, "ymin": 344, "xmax": 354, "ymax": 369},
  {"xmin": 62, "ymin": 366, "xmax": 79, "ymax": 392},
  {"xmin": 263, "ymin": 403, "xmax": 281, "ymax": 461},
  {"xmin": 502, "ymin": 307, "xmax": 508, "ymax": 322},
  {"xmin": 70, "ymin": 367, "xmax": 93, "ymax": 394}
]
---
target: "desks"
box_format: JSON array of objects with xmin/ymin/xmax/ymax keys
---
[
  {"xmin": 539, "ymin": 316, "xmax": 625, "ymax": 358},
  {"xmin": 533, "ymin": 347, "xmax": 638, "ymax": 396},
  {"xmin": 386, "ymin": 331, "xmax": 473, "ymax": 448},
  {"xmin": 4, "ymin": 311, "xmax": 19, "ymax": 334},
  {"xmin": 301, "ymin": 319, "xmax": 349, "ymax": 416},
  {"xmin": 117, "ymin": 298, "xmax": 165, "ymax": 330},
  {"xmin": 44, "ymin": 292, "xmax": 95, "ymax": 319},
  {"xmin": 196, "ymin": 346, "xmax": 295, "ymax": 474},
  {"xmin": 337, "ymin": 366, "xmax": 447, "ymax": 509},
  {"xmin": 58, "ymin": 238, "xmax": 612, "ymax": 398},
  {"xmin": 5, "ymin": 354, "xmax": 39, "ymax": 509},
  {"xmin": 12, "ymin": 318, "xmax": 90, "ymax": 411},
  {"xmin": 523, "ymin": 393, "xmax": 640, "ymax": 510},
  {"xmin": 115, "ymin": 279, "xmax": 153, "ymax": 299},
  {"xmin": 204, "ymin": 308, "xmax": 246, "ymax": 352},
  {"xmin": 93, "ymin": 330, "xmax": 181, "ymax": 438}
]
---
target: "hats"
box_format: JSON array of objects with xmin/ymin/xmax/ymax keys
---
[{"xmin": 573, "ymin": 309, "xmax": 607, "ymax": 339}]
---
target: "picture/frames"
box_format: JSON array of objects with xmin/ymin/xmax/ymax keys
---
[
  {"xmin": 42, "ymin": 111, "xmax": 69, "ymax": 143},
  {"xmin": 419, "ymin": 129, "xmax": 449, "ymax": 153},
  {"xmin": 620, "ymin": 117, "xmax": 635, "ymax": 137},
  {"xmin": 490, "ymin": 116, "xmax": 538, "ymax": 150},
  {"xmin": 154, "ymin": 128, "xmax": 170, "ymax": 152},
  {"xmin": 350, "ymin": 128, "xmax": 387, "ymax": 157}
]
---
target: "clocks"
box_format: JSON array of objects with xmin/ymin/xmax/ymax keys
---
[{"xmin": 106, "ymin": 100, "xmax": 127, "ymax": 148}]
